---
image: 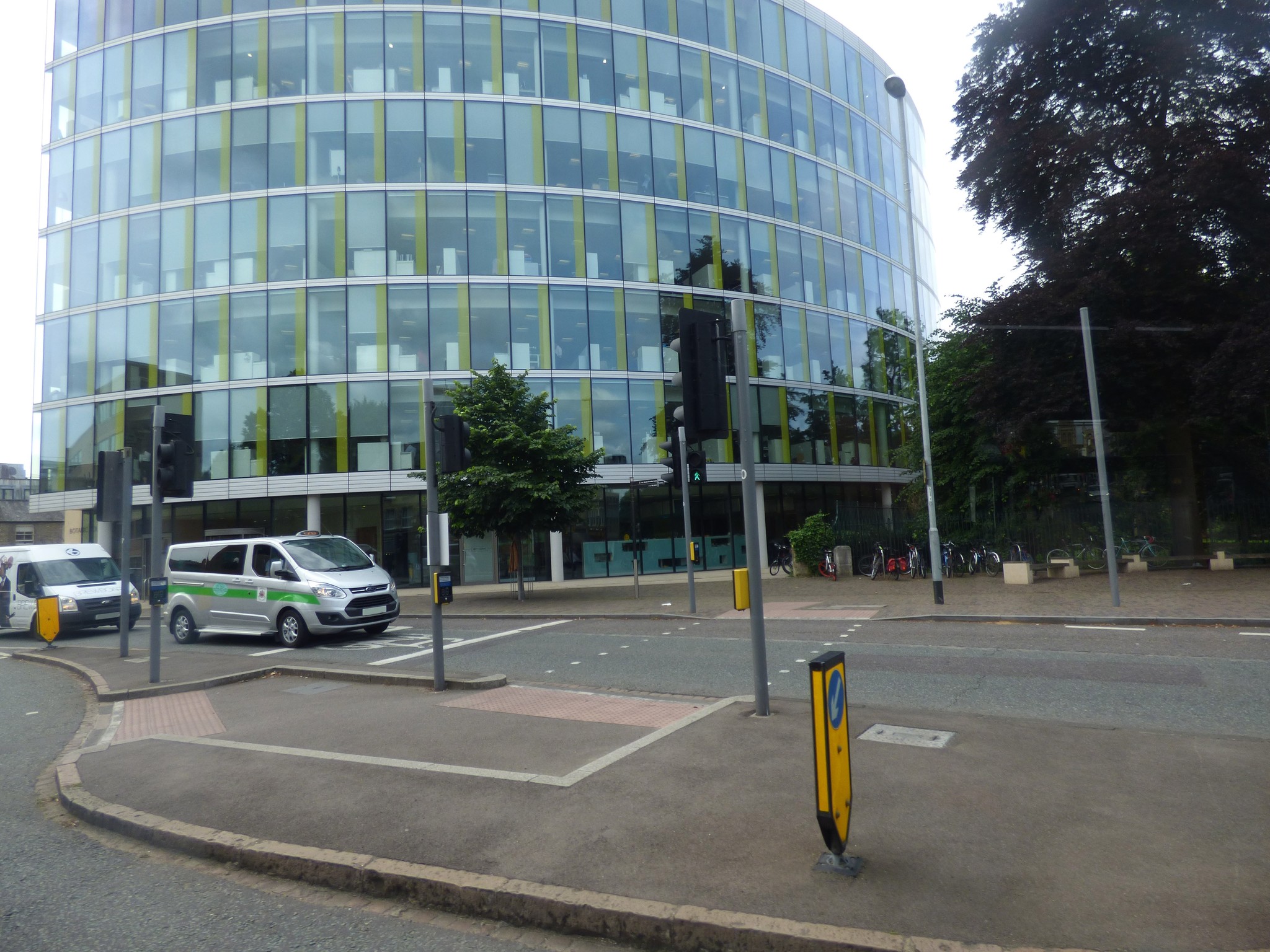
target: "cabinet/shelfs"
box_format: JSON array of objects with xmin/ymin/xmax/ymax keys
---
[
  {"xmin": 213, "ymin": 77, "xmax": 272, "ymax": 103},
  {"xmin": 683, "ymin": 264, "xmax": 718, "ymax": 288},
  {"xmin": 732, "ymin": 273, "xmax": 857, "ymax": 314},
  {"xmin": 616, "ymin": 88, "xmax": 677, "ymax": 117},
  {"xmin": 431, "ymin": 68, "xmax": 454, "ymax": 92},
  {"xmin": 633, "ymin": 259, "xmax": 675, "ymax": 284},
  {"xmin": 481, "ymin": 73, "xmax": 519, "ymax": 95},
  {"xmin": 586, "ymin": 253, "xmax": 598, "ymax": 278},
  {"xmin": 112, "ymin": 343, "xmax": 459, "ymax": 479},
  {"xmin": 492, "ymin": 250, "xmax": 539, "ymax": 276},
  {"xmin": 206, "ymin": 257, "xmax": 256, "ymax": 287},
  {"xmin": 747, "ymin": 114, "xmax": 762, "ymax": 137},
  {"xmin": 443, "ymin": 248, "xmax": 456, "ymax": 275},
  {"xmin": 493, "ymin": 341, "xmax": 867, "ymax": 467},
  {"xmin": 684, "ymin": 98, "xmax": 706, "ymax": 123},
  {"xmin": 579, "ymin": 77, "xmax": 590, "ymax": 103},
  {"xmin": 777, "ymin": 130, "xmax": 849, "ymax": 169},
  {"xmin": 303, "ymin": 249, "xmax": 416, "ymax": 280},
  {"xmin": 354, "ymin": 68, "xmax": 398, "ymax": 92}
]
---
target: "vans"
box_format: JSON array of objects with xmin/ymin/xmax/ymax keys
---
[
  {"xmin": 164, "ymin": 529, "xmax": 401, "ymax": 645},
  {"xmin": 0, "ymin": 543, "xmax": 143, "ymax": 639}
]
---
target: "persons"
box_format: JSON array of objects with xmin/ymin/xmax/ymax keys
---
[
  {"xmin": 0, "ymin": 563, "xmax": 11, "ymax": 627},
  {"xmin": 265, "ymin": 550, "xmax": 285, "ymax": 577}
]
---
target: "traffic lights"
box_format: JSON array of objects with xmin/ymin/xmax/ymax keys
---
[
  {"xmin": 688, "ymin": 450, "xmax": 708, "ymax": 486},
  {"xmin": 155, "ymin": 440, "xmax": 181, "ymax": 492},
  {"xmin": 658, "ymin": 429, "xmax": 681, "ymax": 489},
  {"xmin": 440, "ymin": 414, "xmax": 473, "ymax": 475},
  {"xmin": 670, "ymin": 306, "xmax": 729, "ymax": 448}
]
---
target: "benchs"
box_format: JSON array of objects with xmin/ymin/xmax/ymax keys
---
[{"xmin": 1002, "ymin": 552, "xmax": 1270, "ymax": 585}]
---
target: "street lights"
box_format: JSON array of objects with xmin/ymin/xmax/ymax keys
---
[{"xmin": 884, "ymin": 74, "xmax": 948, "ymax": 607}]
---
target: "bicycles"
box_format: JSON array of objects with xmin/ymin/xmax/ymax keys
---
[
  {"xmin": 818, "ymin": 548, "xmax": 838, "ymax": 581},
  {"xmin": 1047, "ymin": 534, "xmax": 1107, "ymax": 569},
  {"xmin": 769, "ymin": 544, "xmax": 788, "ymax": 576},
  {"xmin": 856, "ymin": 541, "xmax": 890, "ymax": 581},
  {"xmin": 782, "ymin": 548, "xmax": 794, "ymax": 575},
  {"xmin": 886, "ymin": 546, "xmax": 903, "ymax": 580},
  {"xmin": 1005, "ymin": 537, "xmax": 1035, "ymax": 568},
  {"xmin": 1102, "ymin": 534, "xmax": 1169, "ymax": 568},
  {"xmin": 901, "ymin": 540, "xmax": 928, "ymax": 579},
  {"xmin": 941, "ymin": 539, "xmax": 1002, "ymax": 579}
]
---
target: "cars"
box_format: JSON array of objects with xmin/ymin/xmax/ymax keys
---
[{"xmin": 1080, "ymin": 484, "xmax": 1112, "ymax": 502}]
